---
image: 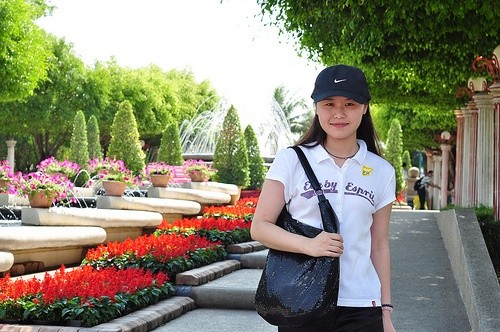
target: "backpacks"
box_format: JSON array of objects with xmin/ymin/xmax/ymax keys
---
[{"xmin": 414, "ymin": 177, "xmax": 423, "ymax": 190}]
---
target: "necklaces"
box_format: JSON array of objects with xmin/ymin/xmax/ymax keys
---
[{"xmin": 322, "ymin": 143, "xmax": 360, "ymax": 159}]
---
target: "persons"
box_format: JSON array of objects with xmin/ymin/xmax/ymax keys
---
[
  {"xmin": 250, "ymin": 65, "xmax": 396, "ymax": 332},
  {"xmin": 417, "ymin": 170, "xmax": 455, "ymax": 210}
]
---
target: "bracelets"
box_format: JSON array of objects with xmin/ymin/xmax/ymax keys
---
[{"xmin": 381, "ymin": 304, "xmax": 393, "ymax": 311}]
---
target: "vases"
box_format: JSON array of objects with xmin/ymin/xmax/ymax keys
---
[
  {"xmin": 102, "ymin": 180, "xmax": 126, "ymax": 197},
  {"xmin": 28, "ymin": 188, "xmax": 54, "ymax": 208},
  {"xmin": 0, "ymin": 178, "xmax": 9, "ymax": 188},
  {"xmin": 150, "ymin": 174, "xmax": 170, "ymax": 187},
  {"xmin": 191, "ymin": 171, "xmax": 206, "ymax": 182}
]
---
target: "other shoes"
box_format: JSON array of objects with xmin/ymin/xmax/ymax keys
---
[{"xmin": 420, "ymin": 207, "xmax": 425, "ymax": 210}]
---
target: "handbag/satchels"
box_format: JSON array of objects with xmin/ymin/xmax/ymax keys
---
[{"xmin": 253, "ymin": 205, "xmax": 341, "ymax": 327}]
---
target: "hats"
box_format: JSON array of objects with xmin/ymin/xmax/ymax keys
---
[{"xmin": 310, "ymin": 64, "xmax": 371, "ymax": 104}]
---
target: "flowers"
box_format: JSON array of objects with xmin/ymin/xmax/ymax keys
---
[{"xmin": 0, "ymin": 156, "xmax": 210, "ymax": 203}]
